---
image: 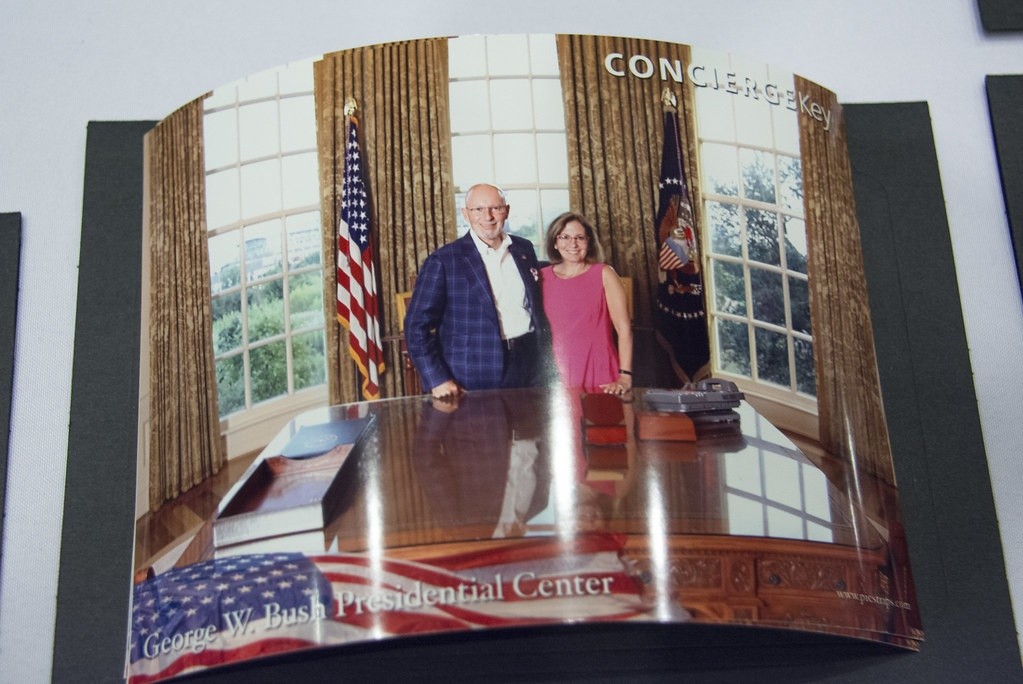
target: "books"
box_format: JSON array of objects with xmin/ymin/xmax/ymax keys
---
[{"xmin": 279, "ymin": 415, "xmax": 377, "ymax": 458}]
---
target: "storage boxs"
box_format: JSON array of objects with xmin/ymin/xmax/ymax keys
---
[{"xmin": 636, "ymin": 410, "xmax": 696, "ymax": 442}]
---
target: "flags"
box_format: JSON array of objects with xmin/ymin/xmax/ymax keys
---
[
  {"xmin": 336, "ymin": 114, "xmax": 386, "ymax": 401},
  {"xmin": 648, "ymin": 105, "xmax": 711, "ymax": 389},
  {"xmin": 124, "ymin": 532, "xmax": 659, "ymax": 684}
]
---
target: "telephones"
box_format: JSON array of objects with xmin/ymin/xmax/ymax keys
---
[{"xmin": 644, "ymin": 377, "xmax": 746, "ymax": 413}]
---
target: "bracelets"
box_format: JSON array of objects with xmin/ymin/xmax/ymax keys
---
[{"xmin": 618, "ymin": 369, "xmax": 633, "ymax": 376}]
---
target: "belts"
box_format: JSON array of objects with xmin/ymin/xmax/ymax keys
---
[{"xmin": 503, "ymin": 333, "xmax": 537, "ymax": 351}]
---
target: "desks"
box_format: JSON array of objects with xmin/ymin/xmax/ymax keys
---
[{"xmin": 135, "ymin": 385, "xmax": 889, "ymax": 640}]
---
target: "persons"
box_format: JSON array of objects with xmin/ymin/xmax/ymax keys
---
[
  {"xmin": 404, "ymin": 183, "xmax": 551, "ymax": 399},
  {"xmin": 540, "ymin": 212, "xmax": 634, "ymax": 395},
  {"xmin": 565, "ymin": 396, "xmax": 636, "ymax": 532},
  {"xmin": 415, "ymin": 390, "xmax": 553, "ymax": 539}
]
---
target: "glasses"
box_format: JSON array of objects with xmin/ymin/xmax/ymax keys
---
[
  {"xmin": 466, "ymin": 206, "xmax": 506, "ymax": 216},
  {"xmin": 556, "ymin": 235, "xmax": 590, "ymax": 243}
]
